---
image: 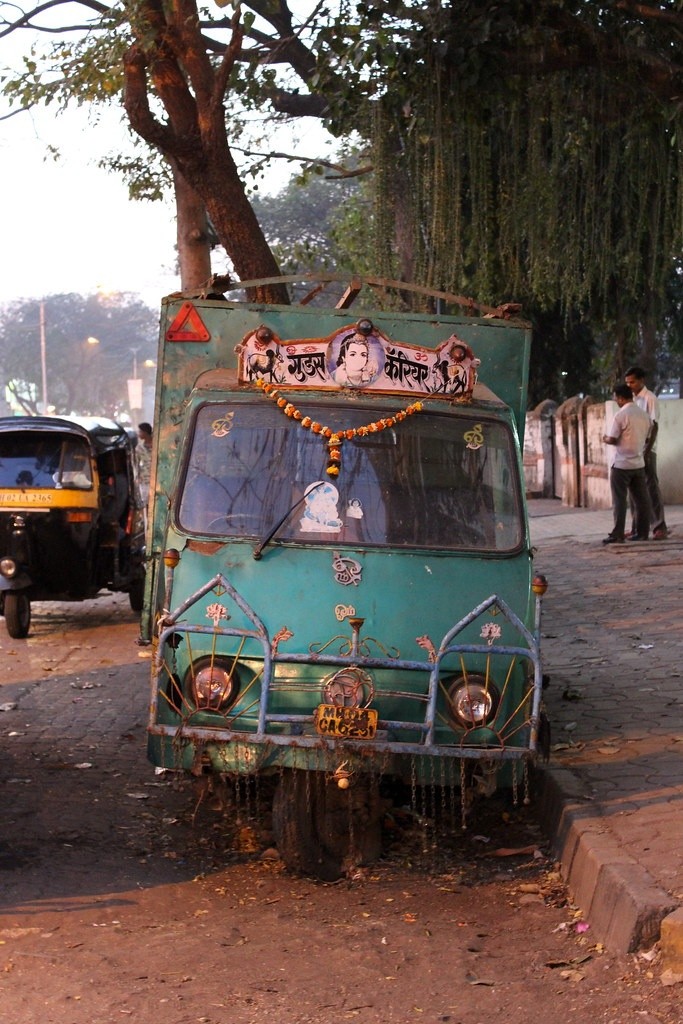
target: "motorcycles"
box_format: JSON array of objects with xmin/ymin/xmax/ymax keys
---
[{"xmin": 0, "ymin": 416, "xmax": 145, "ymax": 640}]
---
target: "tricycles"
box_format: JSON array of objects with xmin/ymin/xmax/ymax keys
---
[{"xmin": 133, "ymin": 269, "xmax": 550, "ymax": 881}]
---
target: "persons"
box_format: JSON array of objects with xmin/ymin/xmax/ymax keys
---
[
  {"xmin": 135, "ymin": 422, "xmax": 154, "ymax": 518},
  {"xmin": 601, "ymin": 384, "xmax": 655, "ymax": 544},
  {"xmin": 330, "ymin": 333, "xmax": 379, "ymax": 389},
  {"xmin": 623, "ymin": 366, "xmax": 668, "ymax": 541}
]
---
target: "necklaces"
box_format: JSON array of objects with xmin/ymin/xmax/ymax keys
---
[{"xmin": 347, "ymin": 376, "xmax": 362, "ymax": 386}]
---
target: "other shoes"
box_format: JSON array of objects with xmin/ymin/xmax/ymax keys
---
[
  {"xmin": 628, "ymin": 533, "xmax": 648, "ymax": 541},
  {"xmin": 653, "ymin": 529, "xmax": 667, "ymax": 539},
  {"xmin": 601, "ymin": 532, "xmax": 625, "ymax": 544},
  {"xmin": 624, "ymin": 532, "xmax": 633, "ymax": 538}
]
